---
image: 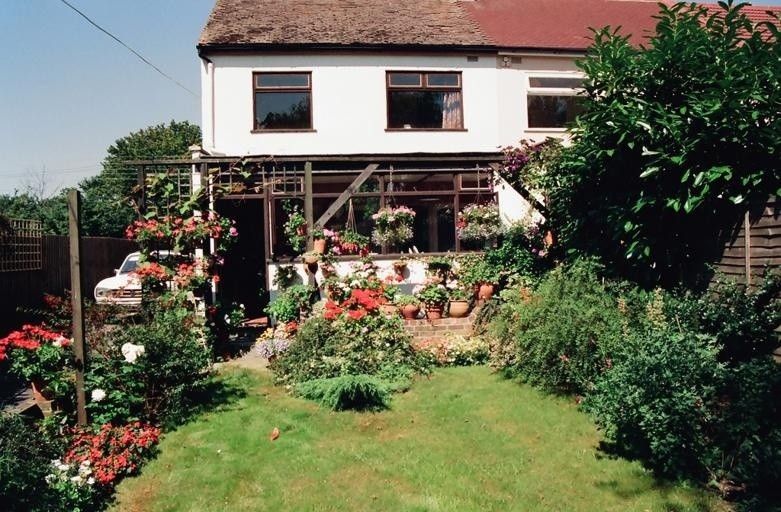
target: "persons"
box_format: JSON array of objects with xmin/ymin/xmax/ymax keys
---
[{"xmin": 207, "ymin": 254, "xmax": 228, "ymax": 303}]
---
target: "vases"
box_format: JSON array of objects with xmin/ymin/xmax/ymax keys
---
[
  {"xmin": 31, "ymin": 381, "xmax": 50, "ymax": 401},
  {"xmin": 449, "ymin": 301, "xmax": 469, "ymax": 318},
  {"xmin": 426, "ymin": 304, "xmax": 443, "ymax": 319}
]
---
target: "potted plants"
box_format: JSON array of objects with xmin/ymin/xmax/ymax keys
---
[{"xmin": 398, "ymin": 294, "xmax": 421, "ymax": 320}]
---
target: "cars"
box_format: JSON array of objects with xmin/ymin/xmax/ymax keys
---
[{"xmin": 94, "ymin": 250, "xmax": 182, "ymax": 318}]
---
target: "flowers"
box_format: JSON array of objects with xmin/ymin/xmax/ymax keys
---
[
  {"xmin": 0, "ymin": 322, "xmax": 72, "ymax": 381},
  {"xmin": 274, "ymin": 199, "xmax": 501, "ymax": 307}
]
